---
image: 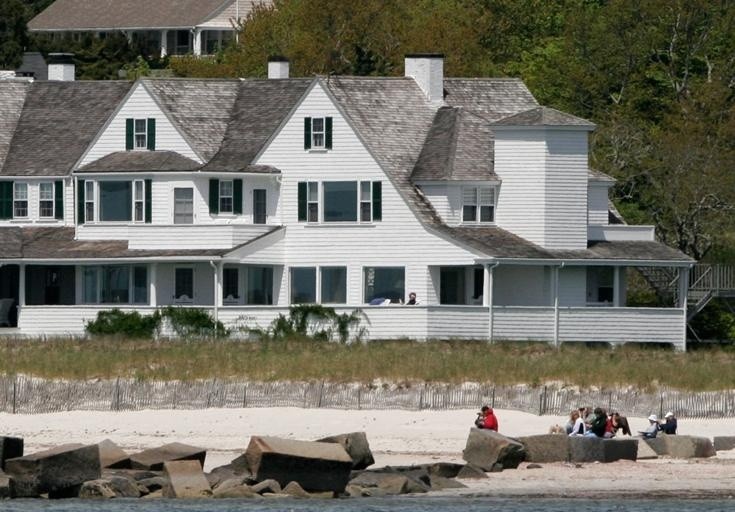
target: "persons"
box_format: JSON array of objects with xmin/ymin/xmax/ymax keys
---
[
  {"xmin": 550, "ymin": 404, "xmax": 632, "ymax": 440},
  {"xmin": 406, "ymin": 292, "xmax": 416, "ymax": 305},
  {"xmin": 475, "ymin": 407, "xmax": 498, "ymax": 432},
  {"xmin": 637, "ymin": 411, "xmax": 677, "ymax": 438}
]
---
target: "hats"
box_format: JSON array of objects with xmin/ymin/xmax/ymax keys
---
[
  {"xmin": 665, "ymin": 412, "xmax": 674, "ymax": 418},
  {"xmin": 649, "ymin": 415, "xmax": 657, "ymax": 422}
]
